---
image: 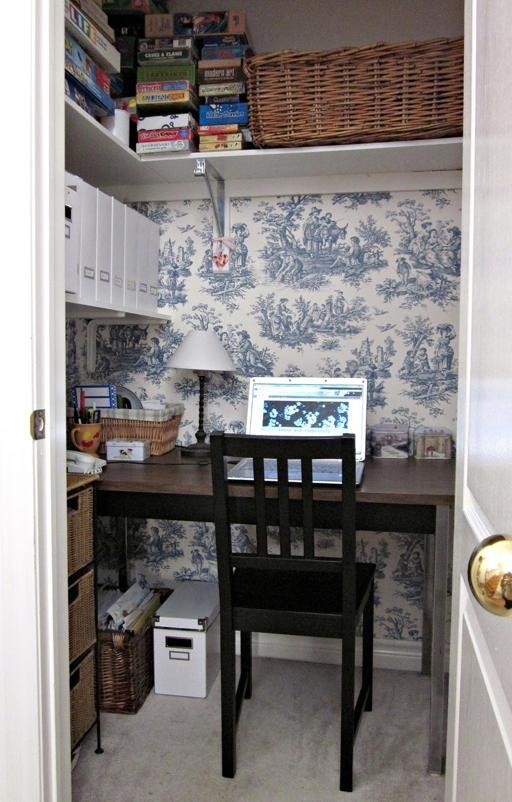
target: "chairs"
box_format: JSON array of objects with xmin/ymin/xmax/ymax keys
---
[{"xmin": 207, "ymin": 430, "xmax": 376, "ymax": 793}]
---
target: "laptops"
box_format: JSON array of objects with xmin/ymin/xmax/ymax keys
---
[{"xmin": 224, "ymin": 377, "xmax": 367, "ymax": 486}]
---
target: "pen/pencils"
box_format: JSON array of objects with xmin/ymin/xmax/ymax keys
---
[{"xmin": 74, "ymin": 390, "xmax": 100, "ymax": 424}]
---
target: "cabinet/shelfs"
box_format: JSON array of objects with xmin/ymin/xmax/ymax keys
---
[{"xmin": 65, "ymin": 468, "xmax": 103, "ymax": 758}]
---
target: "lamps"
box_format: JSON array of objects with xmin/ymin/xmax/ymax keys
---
[{"xmin": 166, "ymin": 329, "xmax": 236, "ymax": 458}]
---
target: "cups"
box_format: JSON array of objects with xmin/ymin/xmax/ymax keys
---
[
  {"xmin": 102, "ymin": 109, "xmax": 130, "ymax": 146},
  {"xmin": 71, "ymin": 422, "xmax": 102, "ymax": 458}
]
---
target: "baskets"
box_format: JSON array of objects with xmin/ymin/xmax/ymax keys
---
[
  {"xmin": 85, "ymin": 403, "xmax": 185, "ymax": 457},
  {"xmin": 94, "ymin": 583, "xmax": 175, "ymax": 716},
  {"xmin": 245, "ymin": 35, "xmax": 469, "ymax": 151}
]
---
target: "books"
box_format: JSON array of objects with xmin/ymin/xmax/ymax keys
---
[{"xmin": 97, "ymin": 581, "xmax": 161, "ymax": 636}]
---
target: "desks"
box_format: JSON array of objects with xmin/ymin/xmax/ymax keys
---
[{"xmin": 85, "ymin": 439, "xmax": 453, "ymax": 785}]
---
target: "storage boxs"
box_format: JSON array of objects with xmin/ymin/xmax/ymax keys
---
[{"xmin": 153, "ymin": 579, "xmax": 220, "ymax": 699}]
